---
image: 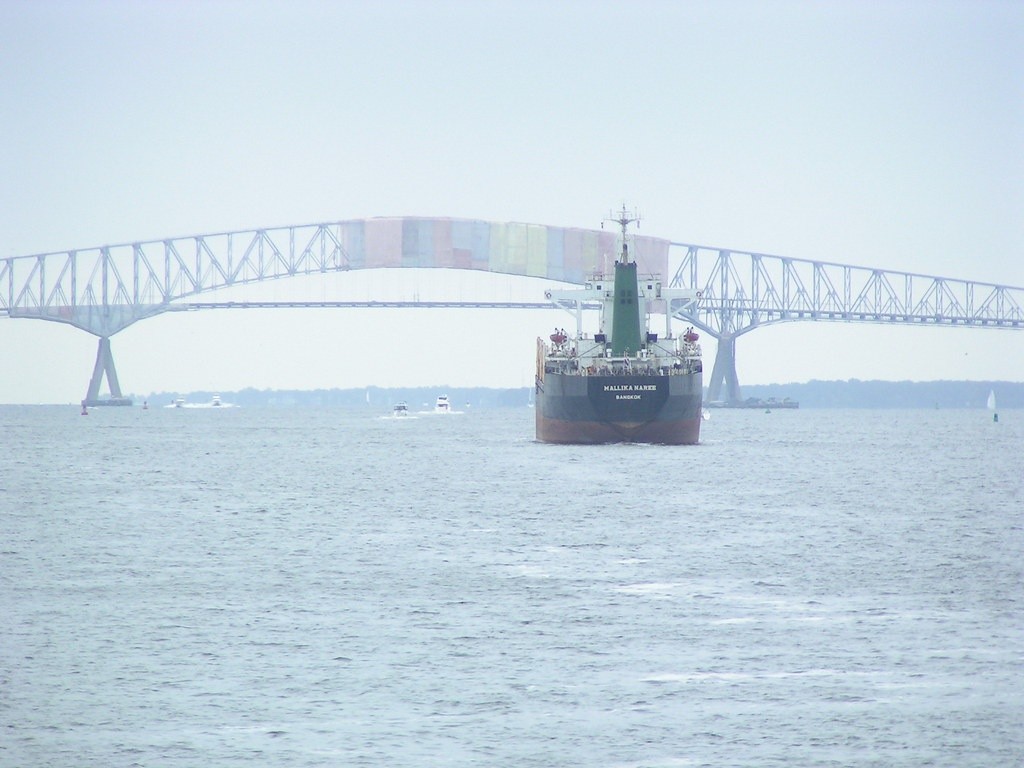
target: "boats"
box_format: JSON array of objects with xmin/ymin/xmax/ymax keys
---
[
  {"xmin": 393, "ymin": 402, "xmax": 407, "ymax": 418},
  {"xmin": 436, "ymin": 394, "xmax": 450, "ymax": 413},
  {"xmin": 212, "ymin": 395, "xmax": 221, "ymax": 407},
  {"xmin": 177, "ymin": 397, "xmax": 185, "ymax": 408},
  {"xmin": 711, "ymin": 393, "xmax": 798, "ymax": 408},
  {"xmin": 535, "ymin": 202, "xmax": 703, "ymax": 444}
]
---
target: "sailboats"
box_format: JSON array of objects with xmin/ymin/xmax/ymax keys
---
[{"xmin": 987, "ymin": 390, "xmax": 998, "ymax": 421}]
